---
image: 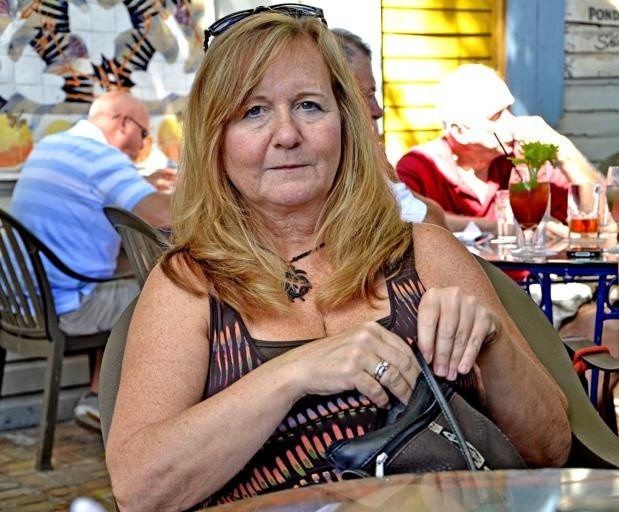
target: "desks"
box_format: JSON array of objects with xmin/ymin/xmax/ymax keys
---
[{"xmin": 455, "ymin": 229, "xmax": 619, "ymax": 407}]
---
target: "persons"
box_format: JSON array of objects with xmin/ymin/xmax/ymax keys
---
[
  {"xmin": 104, "ymin": 3, "xmax": 571, "ymax": 512},
  {"xmin": 396, "ymin": 64, "xmax": 619, "ymax": 281},
  {"xmin": 0, "ymin": 92, "xmax": 176, "ymax": 435},
  {"xmin": 330, "ymin": 28, "xmax": 452, "ymax": 233}
]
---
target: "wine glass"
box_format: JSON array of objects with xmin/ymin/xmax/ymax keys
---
[
  {"xmin": 601, "ymin": 164, "xmax": 619, "ymax": 254},
  {"xmin": 508, "ymin": 165, "xmax": 550, "ymax": 258}
]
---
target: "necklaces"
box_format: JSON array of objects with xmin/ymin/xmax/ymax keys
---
[{"xmin": 255, "ymin": 243, "xmax": 325, "ymax": 301}]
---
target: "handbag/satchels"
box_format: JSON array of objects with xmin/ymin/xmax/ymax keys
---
[{"xmin": 325, "ymin": 336, "xmax": 528, "ymax": 479}]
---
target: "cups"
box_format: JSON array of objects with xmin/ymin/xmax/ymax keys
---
[
  {"xmin": 513, "ymin": 191, "xmax": 551, "ymax": 251},
  {"xmin": 495, "ymin": 189, "xmax": 520, "ymax": 242},
  {"xmin": 565, "ymin": 180, "xmax": 605, "ymax": 259}
]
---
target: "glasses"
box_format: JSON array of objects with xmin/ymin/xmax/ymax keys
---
[
  {"xmin": 112, "ymin": 113, "xmax": 148, "ymax": 138},
  {"xmin": 204, "ymin": 3, "xmax": 327, "ymax": 53}
]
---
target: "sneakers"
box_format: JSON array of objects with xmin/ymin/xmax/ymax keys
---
[{"xmin": 74, "ymin": 390, "xmax": 101, "ymax": 435}]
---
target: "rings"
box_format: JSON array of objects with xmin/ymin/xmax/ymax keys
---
[{"xmin": 371, "ymin": 358, "xmax": 391, "ymax": 381}]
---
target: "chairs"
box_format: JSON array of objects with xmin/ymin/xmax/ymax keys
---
[
  {"xmin": 0, "ymin": 204, "xmax": 136, "ymax": 469},
  {"xmin": 104, "ymin": 206, "xmax": 176, "ymax": 289}
]
